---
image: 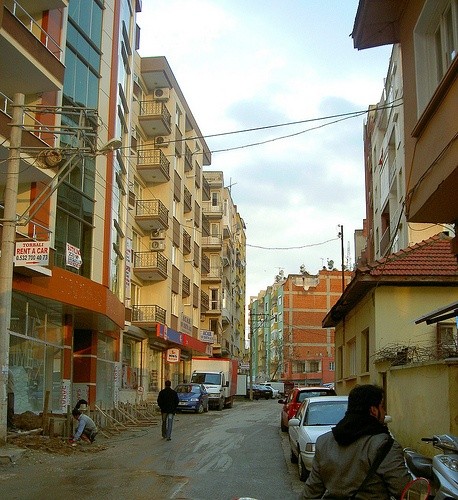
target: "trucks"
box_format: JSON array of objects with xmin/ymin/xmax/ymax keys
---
[{"xmin": 190, "ymin": 358, "xmax": 238, "ymax": 411}]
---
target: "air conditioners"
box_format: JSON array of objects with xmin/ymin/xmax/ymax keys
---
[
  {"xmin": 149, "ymin": 240, "xmax": 165, "ymax": 251},
  {"xmin": 154, "ymin": 87, "xmax": 169, "ymax": 100},
  {"xmin": 155, "ymin": 136, "xmax": 170, "ymax": 147},
  {"xmin": 151, "ymin": 229, "xmax": 166, "ymax": 240}
]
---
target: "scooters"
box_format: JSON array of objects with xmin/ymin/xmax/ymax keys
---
[{"xmin": 402, "ymin": 433, "xmax": 458, "ymax": 500}]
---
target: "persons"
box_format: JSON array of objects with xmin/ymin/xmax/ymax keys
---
[
  {"xmin": 72, "ymin": 409, "xmax": 98, "ymax": 444},
  {"xmin": 297, "ymin": 384, "xmax": 414, "ymax": 500},
  {"xmin": 157, "ymin": 380, "xmax": 179, "ymax": 441}
]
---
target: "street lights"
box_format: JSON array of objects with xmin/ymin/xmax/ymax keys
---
[
  {"xmin": 249, "ymin": 317, "xmax": 275, "ymax": 402},
  {"xmin": 1, "ymin": 138, "xmax": 122, "ymax": 443}
]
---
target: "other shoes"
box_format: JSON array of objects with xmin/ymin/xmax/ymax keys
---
[
  {"xmin": 81, "ymin": 439, "xmax": 91, "ymax": 444},
  {"xmin": 167, "ymin": 438, "xmax": 171, "ymax": 441}
]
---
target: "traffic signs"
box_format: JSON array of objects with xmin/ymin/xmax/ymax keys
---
[{"xmin": 16, "ymin": 241, "xmax": 50, "ymax": 266}]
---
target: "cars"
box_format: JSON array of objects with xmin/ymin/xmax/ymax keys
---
[
  {"xmin": 247, "ymin": 380, "xmax": 392, "ymax": 482},
  {"xmin": 174, "ymin": 383, "xmax": 209, "ymax": 414}
]
